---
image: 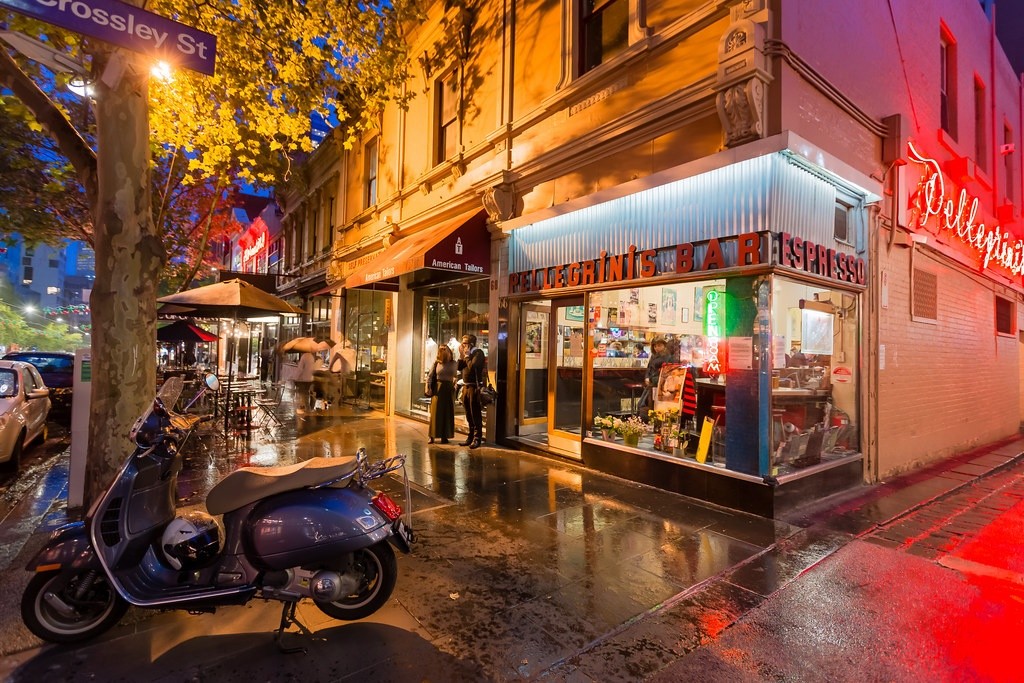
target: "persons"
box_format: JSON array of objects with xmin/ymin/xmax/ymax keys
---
[
  {"xmin": 633, "ymin": 344, "xmax": 648, "ymax": 358},
  {"xmin": 329, "ymin": 340, "xmax": 357, "ymax": 406},
  {"xmin": 293, "ymin": 353, "xmax": 314, "ymax": 421},
  {"xmin": 636, "ymin": 337, "xmax": 674, "ymax": 418},
  {"xmin": 779, "ymin": 347, "xmax": 817, "ymax": 388},
  {"xmin": 426, "ymin": 345, "xmax": 458, "ymax": 444},
  {"xmin": 611, "ymin": 342, "xmax": 627, "ymax": 357},
  {"xmin": 458, "ymin": 334, "xmax": 485, "ymax": 449}
]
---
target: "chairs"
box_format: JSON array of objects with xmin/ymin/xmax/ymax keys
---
[{"xmin": 203, "ymin": 383, "xmax": 285, "ymax": 426}]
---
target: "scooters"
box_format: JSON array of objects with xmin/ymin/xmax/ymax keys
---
[{"xmin": 18, "ymin": 371, "xmax": 415, "ymax": 647}]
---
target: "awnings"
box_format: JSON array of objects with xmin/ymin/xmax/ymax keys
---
[
  {"xmin": 346, "ymin": 203, "xmax": 491, "ymax": 292},
  {"xmin": 311, "ymin": 274, "xmax": 352, "ymax": 297}
]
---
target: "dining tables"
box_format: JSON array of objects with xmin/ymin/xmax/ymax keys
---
[{"xmin": 156, "ymin": 371, "xmax": 266, "ymax": 441}]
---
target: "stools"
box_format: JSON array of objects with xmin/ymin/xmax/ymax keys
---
[
  {"xmin": 773, "ymin": 409, "xmax": 787, "ymax": 450},
  {"xmin": 625, "ymin": 382, "xmax": 643, "ymax": 417},
  {"xmin": 712, "ymin": 404, "xmax": 726, "ymax": 426}
]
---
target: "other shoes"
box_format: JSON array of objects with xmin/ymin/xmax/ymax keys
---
[
  {"xmin": 428, "ymin": 436, "xmax": 433, "ymax": 443},
  {"xmin": 458, "ymin": 438, "xmax": 472, "ymax": 446},
  {"xmin": 440, "ymin": 437, "xmax": 449, "ymax": 444},
  {"xmin": 470, "ymin": 438, "xmax": 481, "ymax": 449}
]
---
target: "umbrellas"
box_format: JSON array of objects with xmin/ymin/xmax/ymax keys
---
[
  {"xmin": 157, "ymin": 304, "xmax": 284, "ymax": 396},
  {"xmin": 156, "ymin": 278, "xmax": 310, "ymax": 442},
  {"xmin": 154, "ymin": 320, "xmax": 222, "ymax": 370},
  {"xmin": 282, "ymin": 337, "xmax": 335, "ymax": 352}
]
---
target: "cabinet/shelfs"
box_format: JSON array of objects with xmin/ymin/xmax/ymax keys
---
[
  {"xmin": 370, "ymin": 358, "xmax": 386, "ymax": 387},
  {"xmin": 565, "ymin": 326, "xmax": 691, "ymax": 358}
]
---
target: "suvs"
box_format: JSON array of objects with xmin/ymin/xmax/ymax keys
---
[{"xmin": 1, "ymin": 351, "xmax": 74, "ymax": 406}]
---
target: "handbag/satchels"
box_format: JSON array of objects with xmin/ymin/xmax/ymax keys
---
[
  {"xmin": 424, "ymin": 361, "xmax": 437, "ymax": 397},
  {"xmin": 475, "ymin": 383, "xmax": 498, "ymax": 407}
]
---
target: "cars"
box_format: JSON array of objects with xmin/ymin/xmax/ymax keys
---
[{"xmin": 0, "ymin": 362, "xmax": 53, "ymax": 465}]
